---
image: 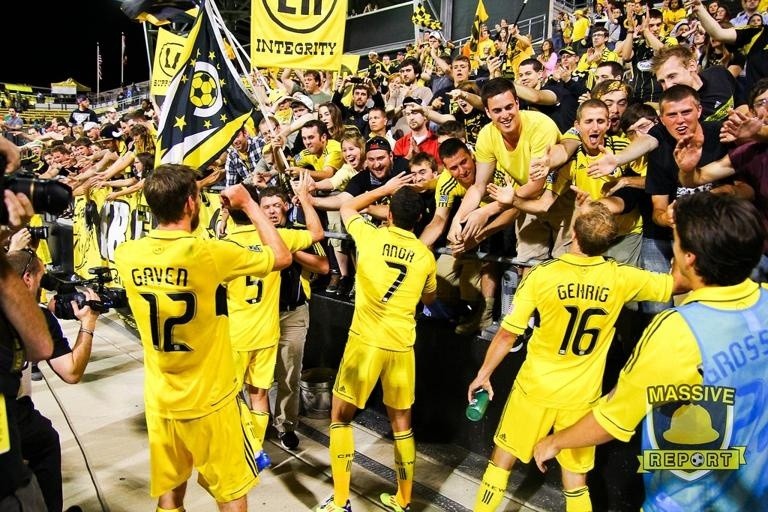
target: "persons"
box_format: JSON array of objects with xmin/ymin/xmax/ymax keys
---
[{"xmin": 0, "ymin": 1, "xmax": 767, "ymax": 512}]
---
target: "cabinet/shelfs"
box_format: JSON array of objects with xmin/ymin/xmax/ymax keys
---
[{"xmin": 466, "ymin": 390, "xmax": 493, "ymax": 422}]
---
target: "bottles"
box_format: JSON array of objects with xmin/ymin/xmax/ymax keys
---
[
  {"xmin": 82, "ymin": 121, "xmax": 99, "ymax": 132},
  {"xmin": 428, "ymin": 31, "xmax": 441, "ymax": 42},
  {"xmin": 403, "ymin": 94, "xmax": 425, "ymax": 107},
  {"xmin": 105, "ymin": 107, "xmax": 116, "ymax": 113},
  {"xmin": 365, "ymin": 137, "xmax": 391, "ymax": 153},
  {"xmin": 559, "ymin": 45, "xmax": 576, "ymax": 56},
  {"xmin": 96, "ymin": 123, "xmax": 124, "ymax": 143}
]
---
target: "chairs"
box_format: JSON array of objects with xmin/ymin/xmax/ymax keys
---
[
  {"xmin": 316, "ymin": 495, "xmax": 353, "ymax": 512},
  {"xmin": 380, "ymin": 493, "xmax": 412, "ymax": 512},
  {"xmin": 481, "ymin": 314, "xmax": 506, "ymax": 339},
  {"xmin": 336, "ymin": 276, "xmax": 353, "ymax": 296},
  {"xmin": 278, "ymin": 431, "xmax": 299, "ymax": 450},
  {"xmin": 325, "ymin": 269, "xmax": 340, "ymax": 293}
]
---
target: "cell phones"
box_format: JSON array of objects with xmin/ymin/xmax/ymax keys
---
[
  {"xmin": 0, "ymin": 177, "xmax": 72, "ymax": 225},
  {"xmin": 25, "ymin": 223, "xmax": 48, "ymax": 242},
  {"xmin": 350, "ymin": 76, "xmax": 364, "ymax": 85}
]
